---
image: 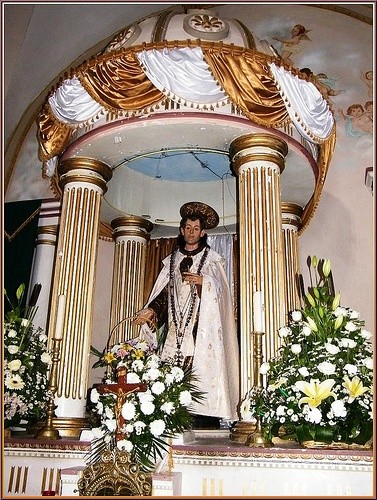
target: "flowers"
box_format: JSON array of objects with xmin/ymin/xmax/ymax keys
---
[
  {"xmin": 82, "ymin": 339, "xmax": 194, "ymax": 454},
  {"xmin": 243, "ymin": 300, "xmax": 373, "ymax": 425},
  {"xmin": 4, "ymin": 317, "xmax": 56, "ymax": 418}
]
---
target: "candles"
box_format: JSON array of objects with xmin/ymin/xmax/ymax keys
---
[
  {"xmin": 55, "ymin": 294, "xmax": 66, "ymax": 339},
  {"xmin": 252, "ymin": 290, "xmax": 264, "ymax": 333}
]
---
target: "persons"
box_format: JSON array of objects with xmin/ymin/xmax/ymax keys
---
[{"xmin": 129, "ymin": 210, "xmax": 239, "ymax": 427}]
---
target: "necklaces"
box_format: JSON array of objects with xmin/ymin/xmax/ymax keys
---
[
  {"xmin": 173, "ymin": 299, "xmax": 191, "ymax": 328},
  {"xmin": 173, "ymin": 276, "xmax": 192, "ymax": 315},
  {"xmin": 169, "ymin": 245, "xmax": 211, "ymax": 367}
]
